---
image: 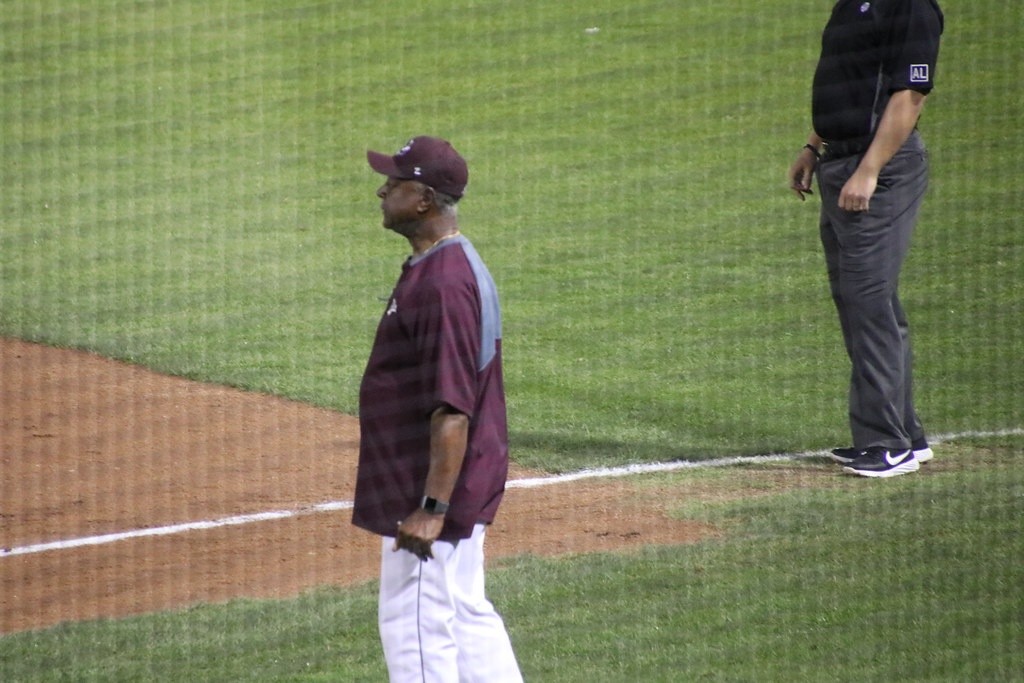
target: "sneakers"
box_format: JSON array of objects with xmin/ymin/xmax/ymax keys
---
[
  {"xmin": 829, "ymin": 436, "xmax": 935, "ymax": 464},
  {"xmin": 843, "ymin": 445, "xmax": 920, "ymax": 478}
]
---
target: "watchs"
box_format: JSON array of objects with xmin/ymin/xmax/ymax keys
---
[{"xmin": 419, "ymin": 495, "xmax": 448, "ymax": 515}]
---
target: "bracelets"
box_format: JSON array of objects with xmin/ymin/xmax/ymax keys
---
[{"xmin": 804, "ymin": 144, "xmax": 821, "ymax": 158}]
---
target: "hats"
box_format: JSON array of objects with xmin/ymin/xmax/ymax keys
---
[{"xmin": 365, "ymin": 135, "xmax": 469, "ymax": 198}]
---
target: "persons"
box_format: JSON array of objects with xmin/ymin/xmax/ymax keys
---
[
  {"xmin": 786, "ymin": 0, "xmax": 945, "ymax": 479},
  {"xmin": 349, "ymin": 138, "xmax": 527, "ymax": 683}
]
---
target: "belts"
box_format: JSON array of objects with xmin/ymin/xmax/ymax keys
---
[{"xmin": 819, "ymin": 138, "xmax": 862, "ymax": 157}]
